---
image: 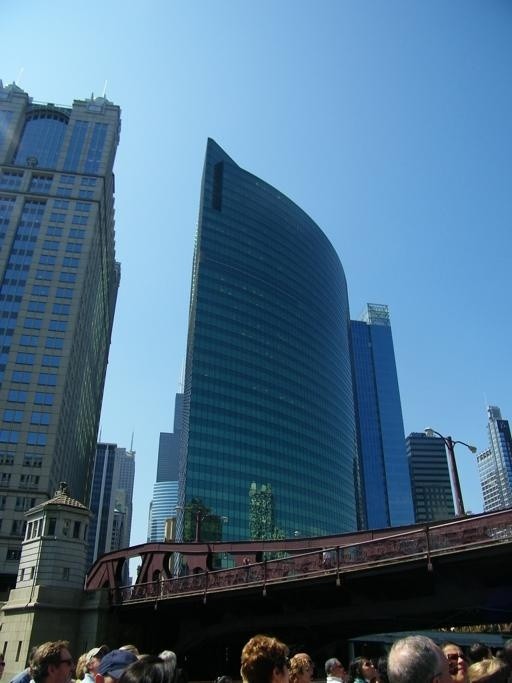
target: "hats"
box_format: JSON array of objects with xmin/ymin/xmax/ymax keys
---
[{"xmin": 85, "ymin": 644, "xmax": 139, "ymax": 679}]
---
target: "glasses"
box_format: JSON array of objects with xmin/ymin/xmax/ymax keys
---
[
  {"xmin": 61, "ymin": 658, "xmax": 74, "ymax": 666},
  {"xmin": 431, "ymin": 663, "xmax": 458, "ymax": 679},
  {"xmin": 448, "ymin": 654, "xmax": 465, "ymax": 663}
]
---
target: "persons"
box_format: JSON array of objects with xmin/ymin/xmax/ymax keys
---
[
  {"xmin": 241, "ymin": 635, "xmax": 512, "ymax": 683},
  {"xmin": 9, "ymin": 641, "xmax": 176, "ymax": 683},
  {"xmin": 241, "ymin": 557, "xmax": 250, "ymax": 582},
  {"xmin": 321, "ymin": 546, "xmax": 331, "ymax": 575}
]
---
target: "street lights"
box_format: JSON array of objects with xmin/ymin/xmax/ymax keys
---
[
  {"xmin": 175, "ymin": 504, "xmax": 229, "ymax": 544},
  {"xmin": 293, "ymin": 529, "xmax": 313, "ymax": 538},
  {"xmin": 424, "ymin": 424, "xmax": 480, "ymax": 519},
  {"xmin": 114, "ymin": 499, "xmax": 128, "ymax": 550}
]
